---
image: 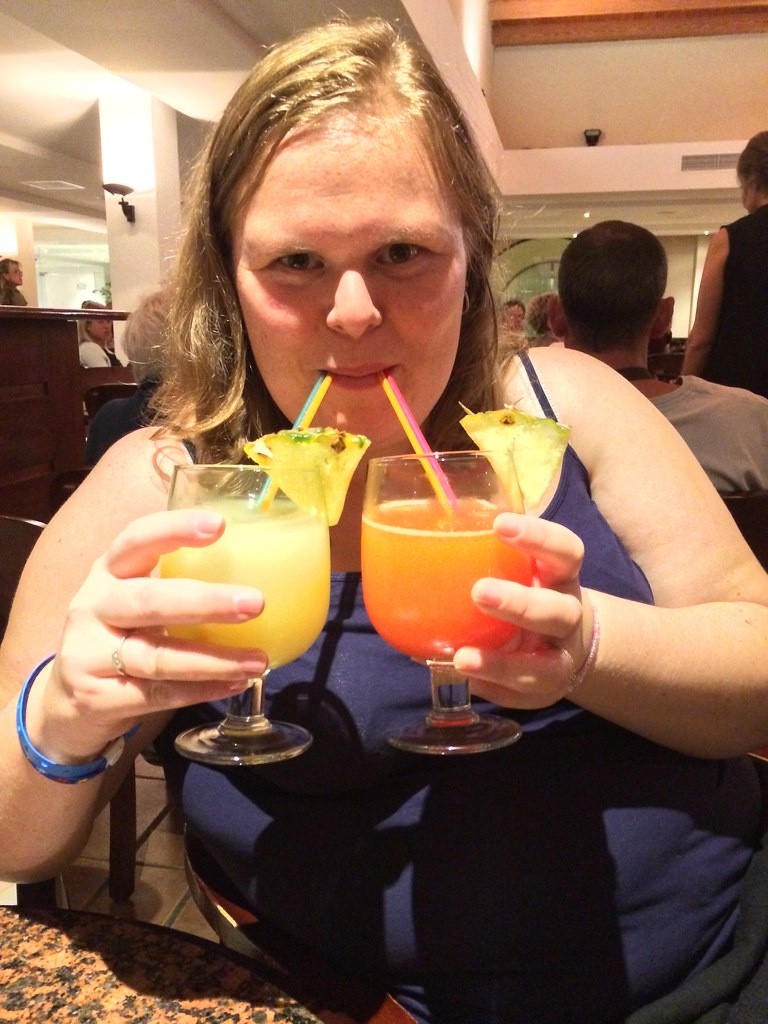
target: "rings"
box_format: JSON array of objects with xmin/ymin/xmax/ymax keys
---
[
  {"xmin": 546, "ymin": 642, "xmax": 576, "ymax": 687},
  {"xmin": 110, "ymin": 632, "xmax": 140, "ymax": 677}
]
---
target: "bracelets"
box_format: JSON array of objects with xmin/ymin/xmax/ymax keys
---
[
  {"xmin": 562, "ymin": 597, "xmax": 600, "ymax": 693},
  {"xmin": 16, "ymin": 653, "xmax": 144, "ymax": 784}
]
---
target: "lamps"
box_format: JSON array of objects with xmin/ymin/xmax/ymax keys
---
[{"xmin": 102, "ymin": 183, "xmax": 135, "ymax": 224}]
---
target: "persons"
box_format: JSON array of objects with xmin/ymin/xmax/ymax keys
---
[
  {"xmin": 679, "ymin": 129, "xmax": 767, "ymax": 399},
  {"xmin": 0, "ymin": 18, "xmax": 768, "ymax": 1022},
  {"xmin": 499, "ymin": 300, "xmax": 535, "ymax": 346},
  {"xmin": 87, "ymin": 290, "xmax": 185, "ymax": 468},
  {"xmin": 0, "ymin": 258, "xmax": 27, "ymax": 307},
  {"xmin": 79, "ymin": 301, "xmax": 124, "ymax": 369},
  {"xmin": 545, "ymin": 221, "xmax": 768, "ymax": 507},
  {"xmin": 524, "ymin": 292, "xmax": 562, "ymax": 349}
]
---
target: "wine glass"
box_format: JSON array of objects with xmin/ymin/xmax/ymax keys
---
[
  {"xmin": 159, "ymin": 465, "xmax": 330, "ymax": 764},
  {"xmin": 361, "ymin": 449, "xmax": 535, "ymax": 754}
]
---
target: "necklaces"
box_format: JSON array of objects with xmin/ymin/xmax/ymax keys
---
[{"xmin": 619, "ymin": 367, "xmax": 652, "ymax": 381}]
